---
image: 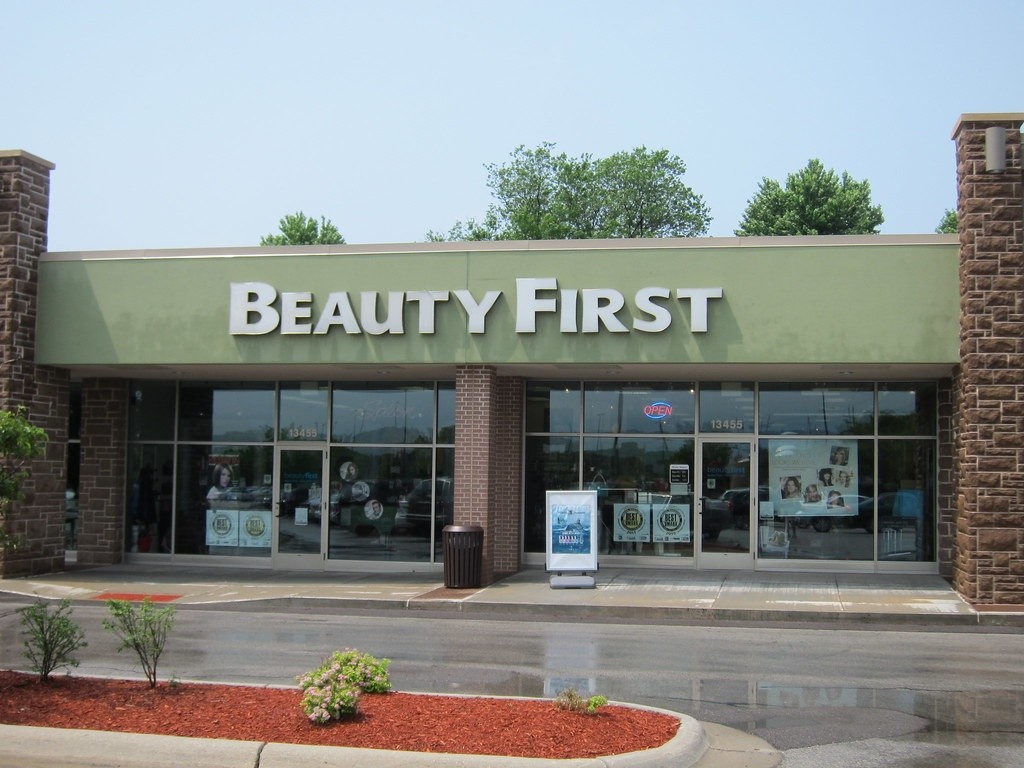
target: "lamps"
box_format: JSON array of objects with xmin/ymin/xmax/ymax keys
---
[{"xmin": 985, "ymin": 126, "xmax": 1006, "ymax": 174}]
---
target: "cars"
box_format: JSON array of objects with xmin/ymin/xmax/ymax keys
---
[
  {"xmin": 219, "ymin": 475, "xmax": 421, "ymax": 529},
  {"xmin": 391, "ymin": 475, "xmax": 453, "ymax": 540},
  {"xmin": 686, "ymin": 480, "xmax": 927, "ymax": 541}
]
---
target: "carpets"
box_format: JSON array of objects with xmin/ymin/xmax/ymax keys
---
[{"xmin": 90, "ymin": 594, "xmax": 182, "ymax": 602}]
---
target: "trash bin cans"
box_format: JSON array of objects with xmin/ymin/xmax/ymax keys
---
[{"xmin": 441, "ymin": 525, "xmax": 483, "ymax": 589}]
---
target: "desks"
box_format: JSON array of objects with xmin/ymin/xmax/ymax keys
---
[
  {"xmin": 208, "ymin": 500, "xmax": 273, "ymax": 556},
  {"xmin": 878, "ymin": 515, "xmax": 918, "ymax": 556}
]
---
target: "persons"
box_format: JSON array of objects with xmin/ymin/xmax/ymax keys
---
[
  {"xmin": 368, "ymin": 501, "xmax": 382, "ymax": 519},
  {"xmin": 819, "ymin": 468, "xmax": 833, "ymax": 486},
  {"xmin": 344, "ymin": 463, "xmax": 358, "ymax": 481},
  {"xmin": 805, "ymin": 485, "xmax": 817, "ymax": 501},
  {"xmin": 206, "ymin": 462, "xmax": 237, "ymax": 499},
  {"xmin": 353, "ymin": 484, "xmax": 368, "ymax": 501},
  {"xmin": 779, "ymin": 501, "xmax": 801, "ymax": 538},
  {"xmin": 831, "ymin": 494, "xmax": 839, "ymax": 506},
  {"xmin": 832, "ymin": 447, "xmax": 847, "ymax": 466},
  {"xmin": 836, "ymin": 471, "xmax": 850, "ymax": 487},
  {"xmin": 784, "ymin": 478, "xmax": 800, "ymax": 498}
]
